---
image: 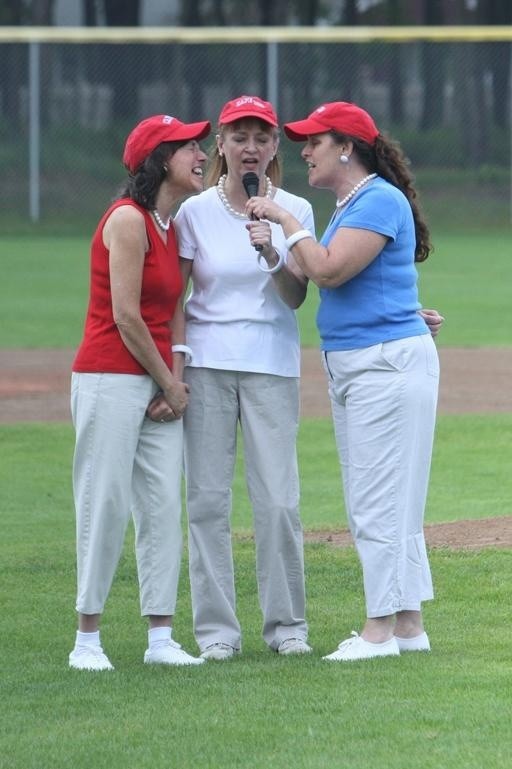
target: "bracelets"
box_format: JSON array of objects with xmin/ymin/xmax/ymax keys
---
[
  {"xmin": 334, "ymin": 172, "xmax": 381, "ymax": 208},
  {"xmin": 285, "ymin": 230, "xmax": 316, "ymax": 252},
  {"xmin": 172, "ymin": 344, "xmax": 194, "ymax": 366},
  {"xmin": 256, "ymin": 246, "xmax": 285, "ymax": 274}
]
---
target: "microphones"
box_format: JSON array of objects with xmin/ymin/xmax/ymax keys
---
[{"xmin": 243, "ymin": 171, "xmax": 266, "ymax": 252}]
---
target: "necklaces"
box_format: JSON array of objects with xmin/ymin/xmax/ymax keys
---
[
  {"xmin": 152, "ymin": 207, "xmax": 173, "ymax": 231},
  {"xmin": 217, "ymin": 173, "xmax": 272, "ymax": 220}
]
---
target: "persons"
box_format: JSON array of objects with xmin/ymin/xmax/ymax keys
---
[
  {"xmin": 173, "ymin": 95, "xmax": 318, "ymax": 664},
  {"xmin": 68, "ymin": 112, "xmax": 212, "ymax": 675},
  {"xmin": 244, "ymin": 98, "xmax": 445, "ymax": 664}
]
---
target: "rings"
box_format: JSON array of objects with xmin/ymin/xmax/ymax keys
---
[{"xmin": 160, "ymin": 419, "xmax": 165, "ymax": 423}]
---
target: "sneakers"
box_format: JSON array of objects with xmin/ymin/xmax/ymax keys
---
[
  {"xmin": 320, "ymin": 629, "xmax": 401, "ymax": 661},
  {"xmin": 390, "ymin": 630, "xmax": 431, "ymax": 654},
  {"xmin": 196, "ymin": 643, "xmax": 244, "ymax": 661},
  {"xmin": 65, "ymin": 646, "xmax": 118, "ymax": 671},
  {"xmin": 275, "ymin": 636, "xmax": 313, "ymax": 656},
  {"xmin": 142, "ymin": 639, "xmax": 207, "ymax": 665}
]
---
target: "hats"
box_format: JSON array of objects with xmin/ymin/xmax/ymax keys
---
[
  {"xmin": 216, "ymin": 93, "xmax": 280, "ymax": 129},
  {"xmin": 121, "ymin": 112, "xmax": 211, "ymax": 174},
  {"xmin": 283, "ymin": 100, "xmax": 380, "ymax": 147}
]
